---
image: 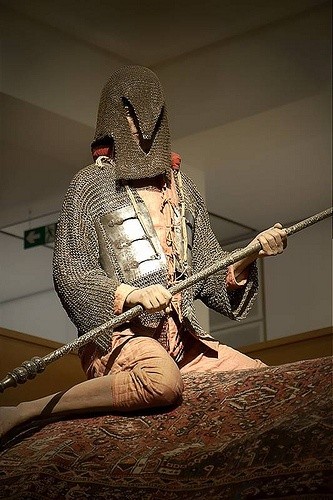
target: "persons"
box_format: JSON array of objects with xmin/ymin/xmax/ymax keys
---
[{"xmin": 0, "ymin": 63, "xmax": 290, "ymax": 440}]
